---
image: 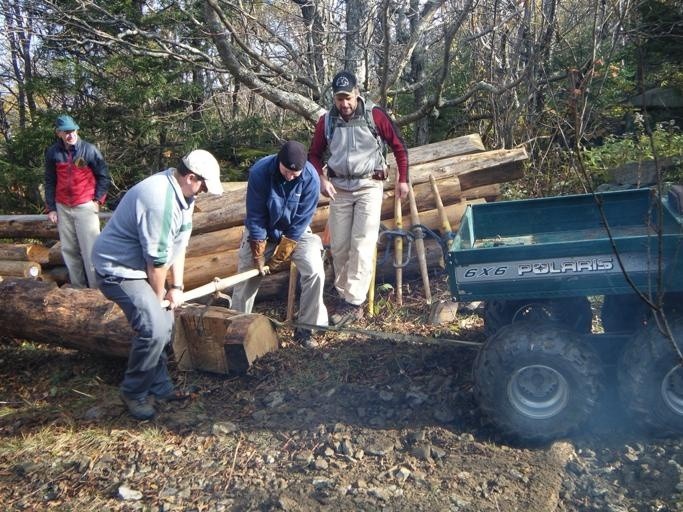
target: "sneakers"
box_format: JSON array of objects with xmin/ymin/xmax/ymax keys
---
[
  {"xmin": 331, "ymin": 300, "xmax": 363, "ymax": 326},
  {"xmin": 121, "ymin": 388, "xmax": 155, "ymax": 420},
  {"xmin": 294, "ymin": 328, "xmax": 318, "ymax": 348},
  {"xmin": 155, "ymin": 391, "xmax": 190, "ymax": 401}
]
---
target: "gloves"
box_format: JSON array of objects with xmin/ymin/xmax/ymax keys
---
[
  {"xmin": 250, "ymin": 240, "xmax": 268, "ymax": 277},
  {"xmin": 265, "ymin": 235, "xmax": 297, "ymax": 272}
]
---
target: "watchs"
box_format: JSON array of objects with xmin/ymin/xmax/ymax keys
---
[
  {"xmin": 92, "ymin": 199, "xmax": 101, "ymax": 206},
  {"xmin": 170, "ymin": 283, "xmax": 185, "ymax": 291}
]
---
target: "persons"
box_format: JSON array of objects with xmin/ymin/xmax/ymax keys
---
[
  {"xmin": 43, "ymin": 113, "xmax": 112, "ymax": 290},
  {"xmin": 228, "ymin": 138, "xmax": 329, "ymax": 351},
  {"xmin": 87, "ymin": 147, "xmax": 226, "ymax": 424},
  {"xmin": 304, "ymin": 70, "xmax": 411, "ymax": 330}
]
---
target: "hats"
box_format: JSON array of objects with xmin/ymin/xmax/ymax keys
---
[
  {"xmin": 183, "ymin": 150, "xmax": 223, "ymax": 196},
  {"xmin": 281, "ymin": 141, "xmax": 307, "ymax": 170},
  {"xmin": 332, "ymin": 71, "xmax": 357, "ymax": 95},
  {"xmin": 57, "ymin": 115, "xmax": 80, "ymax": 131}
]
